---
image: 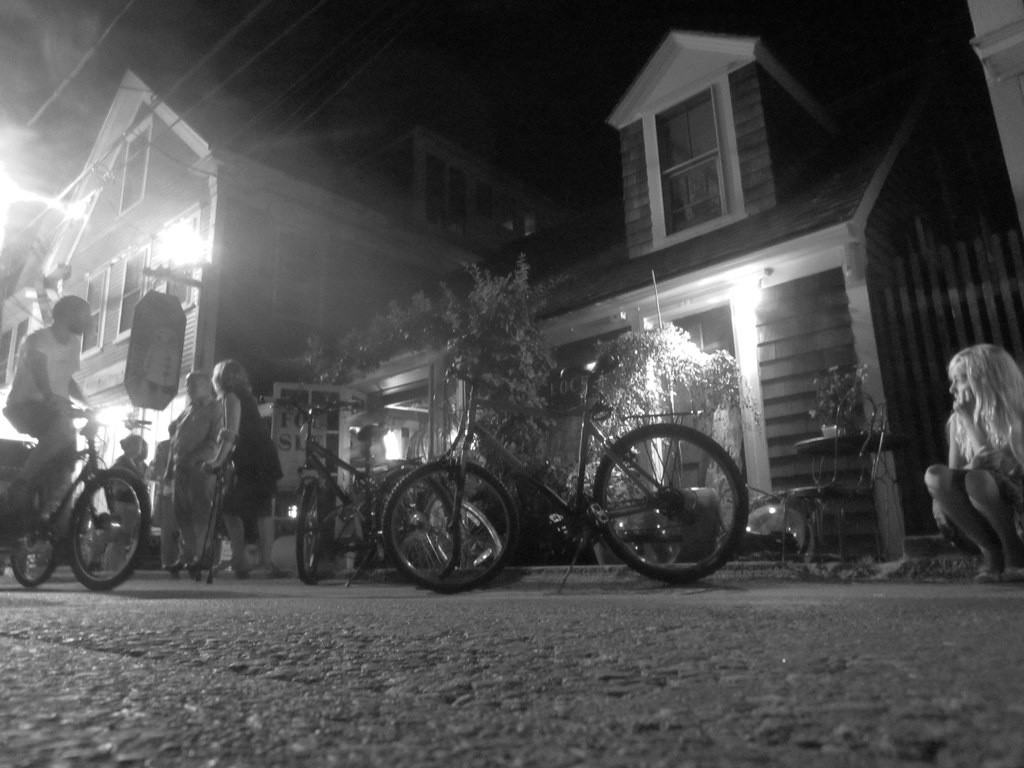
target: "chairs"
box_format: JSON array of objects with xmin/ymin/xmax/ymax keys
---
[{"xmin": 781, "ymin": 392, "xmax": 888, "ymax": 564}]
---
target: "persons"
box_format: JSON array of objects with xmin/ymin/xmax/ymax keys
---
[
  {"xmin": 2, "ymin": 296, "xmax": 97, "ymax": 529},
  {"xmin": 201, "ymin": 360, "xmax": 284, "ymax": 571},
  {"xmin": 104, "ymin": 371, "xmax": 225, "ymax": 570},
  {"xmin": 924, "ymin": 344, "xmax": 1024, "ymax": 582}
]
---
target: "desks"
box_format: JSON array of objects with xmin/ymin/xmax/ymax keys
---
[{"xmin": 793, "ymin": 433, "xmax": 915, "ymax": 562}]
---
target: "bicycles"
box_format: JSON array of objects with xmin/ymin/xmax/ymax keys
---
[
  {"xmin": 259, "ymin": 394, "xmax": 462, "ymax": 585},
  {"xmin": 0, "ymin": 407, "xmax": 152, "ymax": 592},
  {"xmin": 381, "ymin": 353, "xmax": 750, "ymax": 595}
]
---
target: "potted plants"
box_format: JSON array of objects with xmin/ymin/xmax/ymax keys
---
[{"xmin": 579, "ymin": 322, "xmax": 761, "ymax": 567}]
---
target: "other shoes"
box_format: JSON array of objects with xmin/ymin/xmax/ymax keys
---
[
  {"xmin": 233, "ymin": 566, "xmax": 248, "ymax": 579},
  {"xmin": 247, "ymin": 561, "xmax": 273, "ymax": 577},
  {"xmin": 976, "ymin": 567, "xmax": 1024, "ymax": 583},
  {"xmin": 163, "ymin": 561, "xmax": 183, "ymax": 578}
]
